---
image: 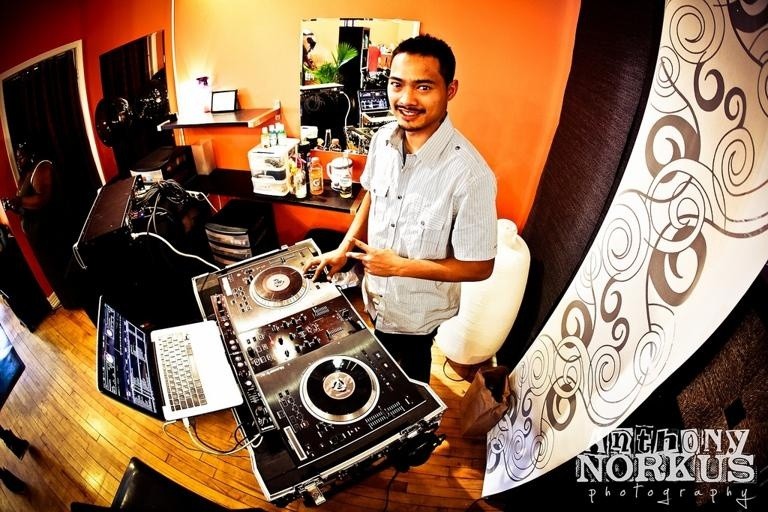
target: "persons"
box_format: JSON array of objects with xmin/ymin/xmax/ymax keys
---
[
  {"xmin": 301, "ymin": 34, "xmax": 499, "ymax": 385},
  {"xmin": 2, "ymin": 142, "xmax": 57, "ymax": 220}
]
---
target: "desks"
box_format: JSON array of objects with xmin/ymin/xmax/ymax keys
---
[{"xmin": 173, "ymin": 165, "xmax": 364, "ymax": 215}]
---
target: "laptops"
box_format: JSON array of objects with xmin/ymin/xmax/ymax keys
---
[
  {"xmin": 357, "ymin": 89, "xmax": 397, "ymax": 123},
  {"xmin": 95, "ymin": 294, "xmax": 244, "ymax": 423}
]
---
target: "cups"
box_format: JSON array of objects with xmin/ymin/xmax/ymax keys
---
[
  {"xmin": 327, "ymin": 157, "xmax": 353, "ymax": 192},
  {"xmin": 344, "ymin": 149, "xmax": 357, "ymax": 154},
  {"xmin": 293, "ymin": 170, "xmax": 307, "ymax": 199}
]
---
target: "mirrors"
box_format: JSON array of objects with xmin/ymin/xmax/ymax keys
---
[{"xmin": 299, "ymin": 16, "xmax": 423, "ymax": 158}]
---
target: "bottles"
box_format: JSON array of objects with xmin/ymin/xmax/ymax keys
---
[
  {"xmin": 308, "ymin": 157, "xmax": 324, "ymax": 195},
  {"xmin": 260, "ymin": 122, "xmax": 286, "ymax": 148},
  {"xmin": 315, "ymin": 129, "xmax": 341, "ymax": 151},
  {"xmin": 347, "ymin": 140, "xmax": 357, "ymax": 150},
  {"xmin": 340, "ymin": 152, "xmax": 352, "ymax": 198}
]
